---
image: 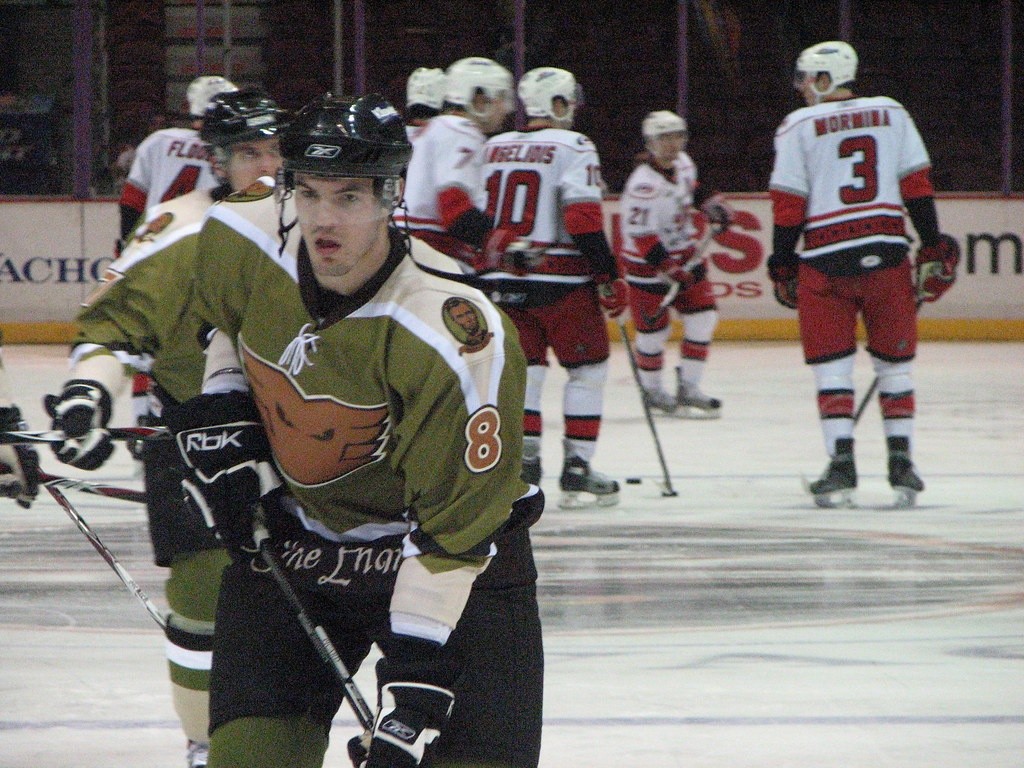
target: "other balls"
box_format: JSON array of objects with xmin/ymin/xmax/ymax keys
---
[{"xmin": 626, "ymin": 478, "xmax": 640, "ymax": 484}]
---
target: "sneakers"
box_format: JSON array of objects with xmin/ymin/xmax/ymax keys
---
[
  {"xmin": 888, "ymin": 435, "xmax": 923, "ymax": 506},
  {"xmin": 643, "ymin": 391, "xmax": 678, "ymax": 417},
  {"xmin": 521, "ymin": 457, "xmax": 540, "ymax": 484},
  {"xmin": 558, "ymin": 439, "xmax": 619, "ymax": 508},
  {"xmin": 811, "ymin": 437, "xmax": 857, "ymax": 508},
  {"xmin": 675, "ymin": 366, "xmax": 721, "ymax": 417}
]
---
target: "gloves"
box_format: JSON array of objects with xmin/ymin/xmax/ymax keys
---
[
  {"xmin": 162, "ymin": 390, "xmax": 287, "ymax": 545},
  {"xmin": 589, "ymin": 257, "xmax": 628, "ymax": 317},
  {"xmin": 696, "ymin": 186, "xmax": 734, "ymax": 224},
  {"xmin": 0, "ymin": 404, "xmax": 40, "ymax": 508},
  {"xmin": 348, "ymin": 649, "xmax": 458, "ymax": 768},
  {"xmin": 484, "ymin": 228, "xmax": 533, "ymax": 274},
  {"xmin": 44, "ymin": 379, "xmax": 114, "ymax": 470},
  {"xmin": 661, "ymin": 259, "xmax": 692, "ymax": 289},
  {"xmin": 769, "ymin": 253, "xmax": 799, "ymax": 308},
  {"xmin": 915, "ymin": 234, "xmax": 958, "ymax": 300}
]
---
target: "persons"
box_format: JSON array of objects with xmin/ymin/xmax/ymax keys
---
[
  {"xmin": 402, "ymin": 58, "xmax": 542, "ymax": 280},
  {"xmin": 472, "ymin": 66, "xmax": 631, "ymax": 509},
  {"xmin": 767, "ymin": 42, "xmax": 950, "ymax": 508},
  {"xmin": 114, "ymin": 73, "xmax": 241, "ymax": 458},
  {"xmin": 48, "ymin": 96, "xmax": 544, "ymax": 766},
  {"xmin": 402, "ymin": 66, "xmax": 447, "ymax": 148},
  {"xmin": 110, "ymin": 89, "xmax": 294, "ymax": 766},
  {"xmin": 623, "ymin": 109, "xmax": 723, "ymax": 418},
  {"xmin": 114, "ymin": 113, "xmax": 166, "ymax": 199}
]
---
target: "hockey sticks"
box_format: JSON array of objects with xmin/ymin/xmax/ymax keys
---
[
  {"xmin": 0, "ymin": 426, "xmax": 172, "ymax": 445},
  {"xmin": 800, "ymin": 376, "xmax": 881, "ymax": 492},
  {"xmin": 248, "ymin": 520, "xmax": 375, "ymax": 739},
  {"xmin": 604, "ymin": 283, "xmax": 678, "ymax": 499},
  {"xmin": 638, "ymin": 220, "xmax": 724, "ymax": 327},
  {"xmin": 34, "ymin": 458, "xmax": 168, "ymax": 635},
  {"xmin": 0, "ymin": 463, "xmax": 147, "ymax": 504},
  {"xmin": 535, "ymin": 184, "xmax": 564, "ymax": 256}
]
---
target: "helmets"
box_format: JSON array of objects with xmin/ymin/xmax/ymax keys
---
[
  {"xmin": 446, "ymin": 56, "xmax": 513, "ymax": 105},
  {"xmin": 186, "ymin": 73, "xmax": 237, "ymax": 116},
  {"xmin": 642, "ymin": 111, "xmax": 686, "ymax": 139},
  {"xmin": 797, "ymin": 41, "xmax": 857, "ymax": 86},
  {"xmin": 405, "ymin": 67, "xmax": 449, "ymax": 110},
  {"xmin": 517, "ymin": 67, "xmax": 576, "ymax": 116},
  {"xmin": 200, "ymin": 86, "xmax": 292, "ymax": 153},
  {"xmin": 278, "ymin": 91, "xmax": 412, "ymax": 187}
]
